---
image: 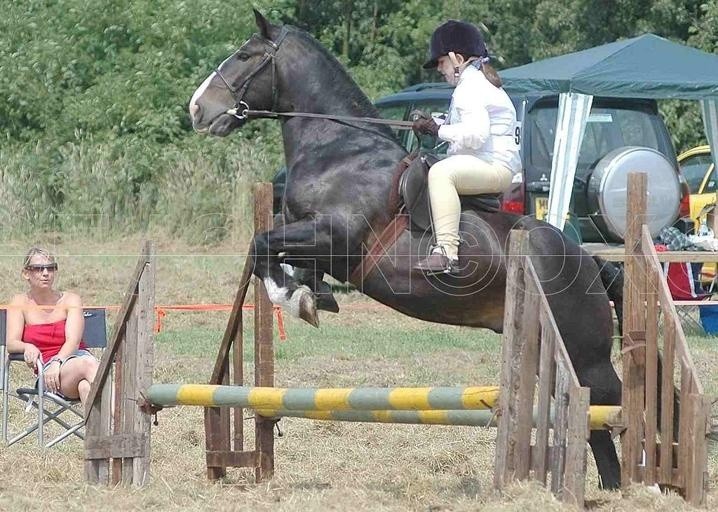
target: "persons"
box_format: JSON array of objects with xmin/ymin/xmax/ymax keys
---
[
  {"xmin": 409, "ymin": 20, "xmax": 524, "ymax": 276},
  {"xmin": 4, "ymin": 245, "xmax": 116, "ymax": 418}
]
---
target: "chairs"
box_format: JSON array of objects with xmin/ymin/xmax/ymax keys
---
[
  {"xmin": 0, "ymin": 305, "xmax": 111, "ymax": 453},
  {"xmin": 651, "ymin": 252, "xmax": 712, "ymax": 338}
]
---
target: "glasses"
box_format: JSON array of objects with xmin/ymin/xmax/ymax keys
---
[{"xmin": 24, "ymin": 262, "xmax": 58, "ymax": 273}]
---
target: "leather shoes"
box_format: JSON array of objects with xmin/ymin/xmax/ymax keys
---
[{"xmin": 411, "ymin": 252, "xmax": 460, "ymax": 276}]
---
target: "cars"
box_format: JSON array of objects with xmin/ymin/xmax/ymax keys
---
[{"xmin": 677, "ymin": 146, "xmax": 717, "ymax": 285}]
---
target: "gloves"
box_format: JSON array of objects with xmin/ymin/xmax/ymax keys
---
[
  {"xmin": 410, "ymin": 108, "xmax": 430, "ymax": 119},
  {"xmin": 413, "ymin": 118, "xmax": 441, "ymax": 137}
]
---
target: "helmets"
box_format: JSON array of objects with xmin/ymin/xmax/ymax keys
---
[{"xmin": 423, "ymin": 19, "xmax": 487, "ymax": 69}]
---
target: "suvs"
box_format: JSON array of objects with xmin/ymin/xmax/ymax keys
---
[{"xmin": 274, "ymin": 92, "xmax": 693, "ymax": 242}]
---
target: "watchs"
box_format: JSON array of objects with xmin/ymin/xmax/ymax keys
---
[{"xmin": 51, "ymin": 356, "xmax": 63, "ymax": 363}]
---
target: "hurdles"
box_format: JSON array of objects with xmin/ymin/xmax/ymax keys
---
[
  {"xmin": 201, "ymin": 172, "xmax": 710, "ymax": 509},
  {"xmin": 78, "ymin": 228, "xmax": 590, "ymax": 511}
]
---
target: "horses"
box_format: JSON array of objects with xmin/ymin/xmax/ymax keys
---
[{"xmin": 186, "ymin": 7, "xmax": 680, "ymax": 491}]
